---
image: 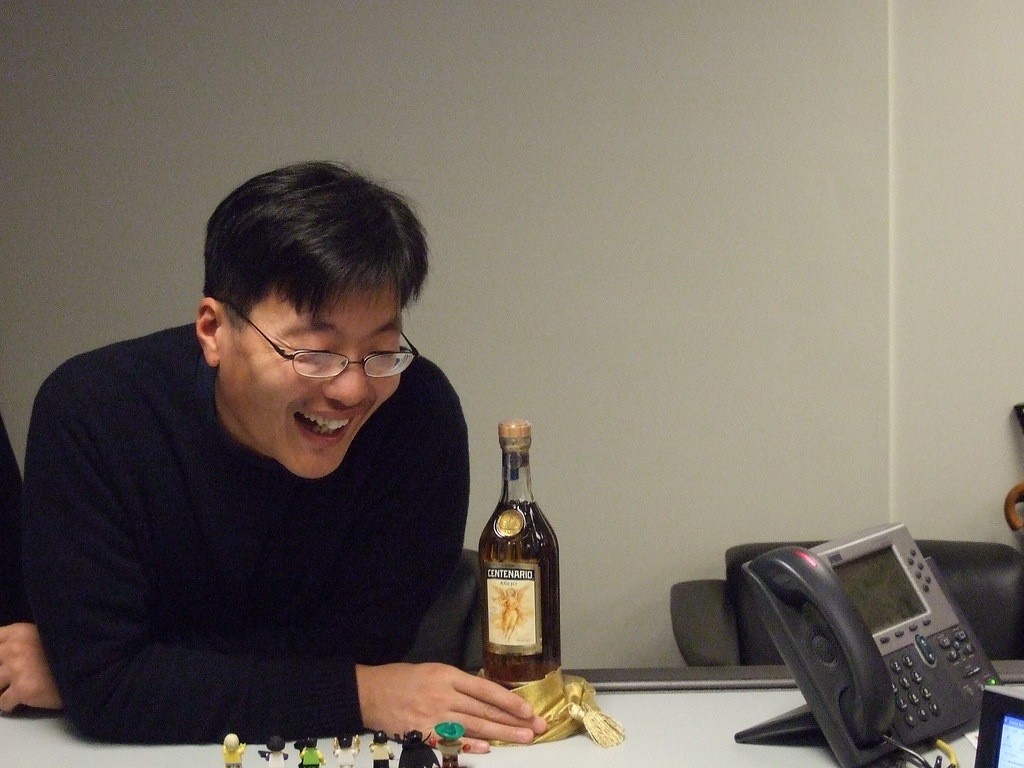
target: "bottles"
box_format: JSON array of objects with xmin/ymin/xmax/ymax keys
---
[{"xmin": 479, "ymin": 419, "xmax": 561, "ymax": 691}]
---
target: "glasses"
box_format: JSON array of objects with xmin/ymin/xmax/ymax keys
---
[{"xmin": 220, "ymin": 300, "xmax": 419, "ymax": 378}]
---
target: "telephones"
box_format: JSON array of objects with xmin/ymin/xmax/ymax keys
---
[{"xmin": 740, "ymin": 522, "xmax": 1005, "ymax": 768}]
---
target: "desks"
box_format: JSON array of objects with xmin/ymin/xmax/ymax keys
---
[{"xmin": 0, "ymin": 671, "xmax": 1024, "ymax": 768}]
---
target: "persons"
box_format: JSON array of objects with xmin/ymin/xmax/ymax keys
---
[
  {"xmin": 331, "ymin": 734, "xmax": 360, "ymax": 768},
  {"xmin": 223, "ymin": 733, "xmax": 247, "ymax": 768},
  {"xmin": 258, "ymin": 736, "xmax": 288, "ymax": 768},
  {"xmin": 299, "ymin": 737, "xmax": 326, "ymax": 768},
  {"xmin": 369, "ymin": 730, "xmax": 394, "ymax": 768},
  {"xmin": 398, "ymin": 730, "xmax": 441, "ymax": 768},
  {"xmin": 0, "ymin": 159, "xmax": 547, "ymax": 753},
  {"xmin": 435, "ymin": 721, "xmax": 465, "ymax": 768}
]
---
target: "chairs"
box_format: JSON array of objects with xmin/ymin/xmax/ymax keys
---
[{"xmin": 668, "ymin": 543, "xmax": 1024, "ymax": 667}]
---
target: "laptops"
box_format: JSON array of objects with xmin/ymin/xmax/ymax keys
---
[{"xmin": 976, "ymin": 687, "xmax": 1024, "ymax": 768}]
